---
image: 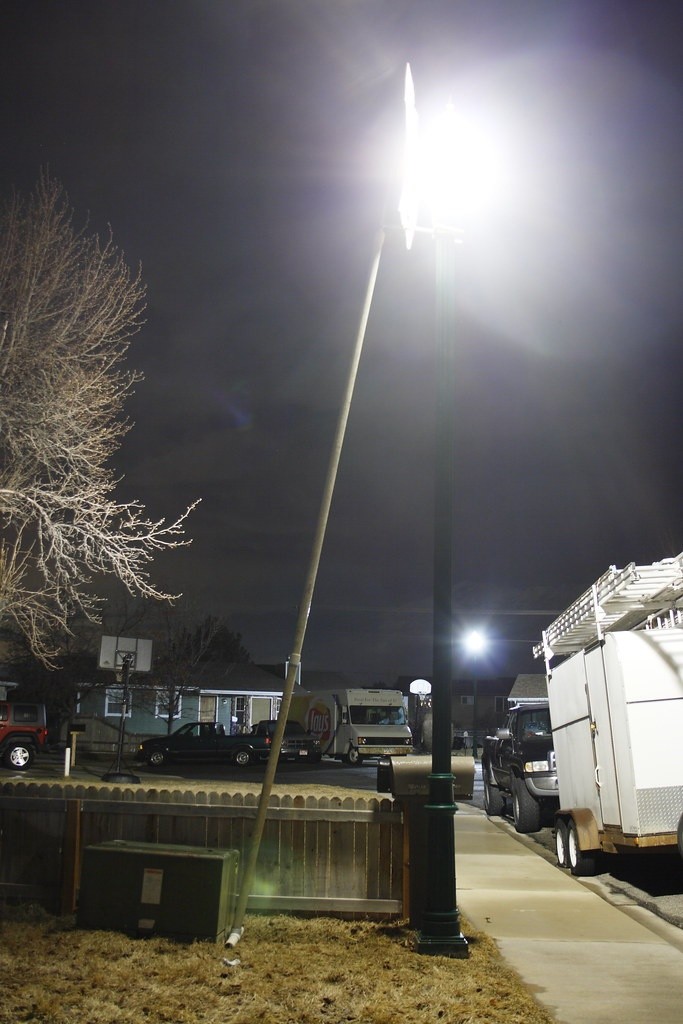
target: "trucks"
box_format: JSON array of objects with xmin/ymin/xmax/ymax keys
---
[{"xmin": 287, "ymin": 689, "xmax": 413, "ymax": 766}]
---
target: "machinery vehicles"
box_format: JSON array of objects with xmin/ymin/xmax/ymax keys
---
[{"xmin": 533, "ymin": 552, "xmax": 683, "ymax": 876}]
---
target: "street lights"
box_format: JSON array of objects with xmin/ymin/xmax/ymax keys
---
[
  {"xmin": 465, "ymin": 632, "xmax": 482, "ymax": 758},
  {"xmin": 414, "ymin": 174, "xmax": 471, "ymax": 959}
]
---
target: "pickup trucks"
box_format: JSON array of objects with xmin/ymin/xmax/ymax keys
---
[
  {"xmin": 250, "ymin": 720, "xmax": 321, "ymax": 764},
  {"xmin": 135, "ymin": 722, "xmax": 288, "ymax": 768}
]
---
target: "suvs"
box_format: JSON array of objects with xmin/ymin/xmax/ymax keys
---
[
  {"xmin": 0, "ymin": 700, "xmax": 48, "ymax": 770},
  {"xmin": 481, "ymin": 702, "xmax": 560, "ymax": 833}
]
